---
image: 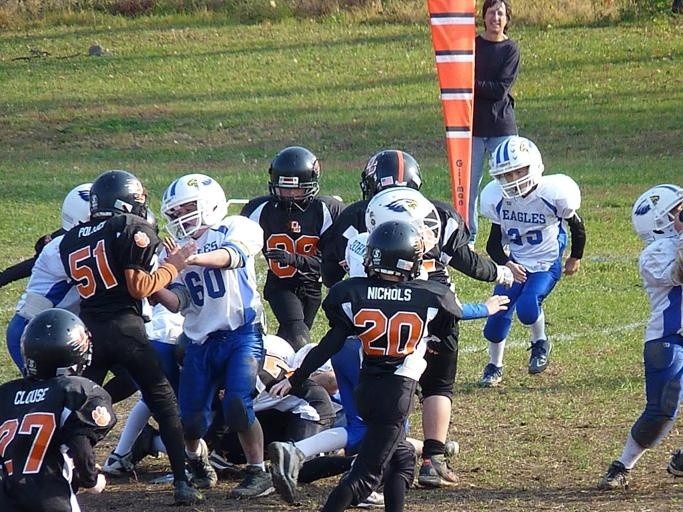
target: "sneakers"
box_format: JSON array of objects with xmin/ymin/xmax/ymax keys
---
[
  {"xmin": 267, "ymin": 440, "xmax": 306, "ymax": 505},
  {"xmin": 171, "ymin": 440, "xmax": 238, "ymax": 507},
  {"xmin": 596, "ymin": 458, "xmax": 632, "ymax": 490},
  {"xmin": 231, "ymin": 462, "xmax": 278, "ymax": 501},
  {"xmin": 356, "ymin": 488, "xmax": 384, "ymax": 507},
  {"xmin": 667, "ymin": 448, "xmax": 683, "ymax": 478},
  {"xmin": 527, "ymin": 334, "xmax": 555, "ymax": 374},
  {"xmin": 101, "ymin": 446, "xmax": 136, "ymax": 476},
  {"xmin": 130, "ymin": 426, "xmax": 160, "ymax": 467},
  {"xmin": 419, "ymin": 440, "xmax": 462, "ymax": 485},
  {"xmin": 478, "ymin": 362, "xmax": 504, "ymax": 388}
]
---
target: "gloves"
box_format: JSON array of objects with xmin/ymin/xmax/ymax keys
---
[{"xmin": 267, "ymin": 245, "xmax": 294, "ymax": 269}]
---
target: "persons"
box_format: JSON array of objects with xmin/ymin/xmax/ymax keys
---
[
  {"xmin": 598, "ymin": 182, "xmax": 683, "ymax": 489},
  {"xmin": 479, "ymin": 136, "xmax": 586, "ymax": 388},
  {"xmin": 0, "ymin": 146, "xmax": 514, "ymax": 512},
  {"xmin": 468, "ymin": 0, "xmax": 519, "ymax": 251}
]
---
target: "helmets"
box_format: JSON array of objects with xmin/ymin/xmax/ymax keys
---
[
  {"xmin": 161, "ymin": 173, "xmax": 228, "ymax": 242},
  {"xmin": 18, "ymin": 309, "xmax": 93, "ymax": 378},
  {"xmin": 60, "ymin": 182, "xmax": 99, "ymax": 231},
  {"xmin": 259, "ymin": 334, "xmax": 295, "ymax": 366},
  {"xmin": 486, "ymin": 134, "xmax": 544, "ymax": 201},
  {"xmin": 295, "ymin": 337, "xmax": 331, "ymax": 374},
  {"xmin": 359, "ymin": 148, "xmax": 440, "ymax": 279},
  {"xmin": 266, "ymin": 144, "xmax": 322, "ymax": 212},
  {"xmin": 89, "ymin": 170, "xmax": 148, "ymax": 219},
  {"xmin": 631, "ymin": 182, "xmax": 683, "ymax": 242}
]
